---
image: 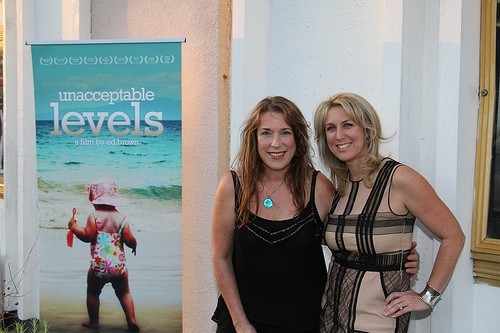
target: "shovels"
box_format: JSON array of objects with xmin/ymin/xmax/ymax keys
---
[{"xmin": 67, "ymin": 208, "xmax": 76, "ymax": 247}]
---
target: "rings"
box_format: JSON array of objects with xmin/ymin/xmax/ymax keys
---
[{"xmin": 399, "ymin": 302, "xmax": 403, "ymax": 310}]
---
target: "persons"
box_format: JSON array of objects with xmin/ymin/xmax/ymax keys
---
[
  {"xmin": 68, "ymin": 181, "xmax": 137, "ymax": 328},
  {"xmin": 210, "ymin": 96, "xmax": 421, "ymax": 333},
  {"xmin": 314, "ymin": 93, "xmax": 466, "ymax": 333}
]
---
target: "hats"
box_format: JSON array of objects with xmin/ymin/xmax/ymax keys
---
[{"xmin": 87, "ymin": 181, "xmax": 118, "ymax": 206}]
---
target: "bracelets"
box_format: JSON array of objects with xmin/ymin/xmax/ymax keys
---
[{"xmin": 418, "ymin": 282, "xmax": 442, "ymax": 308}]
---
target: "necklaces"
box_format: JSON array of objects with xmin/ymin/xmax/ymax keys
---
[{"xmin": 258, "ymin": 174, "xmax": 287, "ymax": 209}]
